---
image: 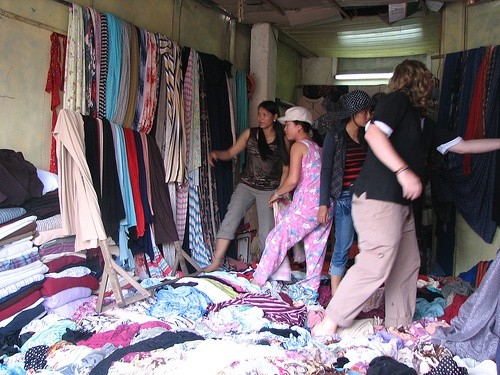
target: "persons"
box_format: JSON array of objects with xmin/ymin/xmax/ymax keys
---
[
  {"xmin": 251, "ymin": 107, "xmax": 335, "ymax": 292},
  {"xmin": 318, "ymin": 90, "xmax": 373, "ymax": 296},
  {"xmin": 200, "ymin": 101, "xmax": 291, "ymax": 272},
  {"xmin": 310, "ymin": 60, "xmax": 500, "ymax": 336}
]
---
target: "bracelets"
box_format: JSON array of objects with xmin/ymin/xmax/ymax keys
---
[
  {"xmin": 274, "ymin": 190, "xmax": 283, "ymax": 198},
  {"xmin": 394, "ymin": 164, "xmax": 409, "ymax": 175}
]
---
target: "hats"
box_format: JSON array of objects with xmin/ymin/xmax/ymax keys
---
[
  {"xmin": 339, "ymin": 90, "xmax": 374, "ymax": 119},
  {"xmin": 277, "ymin": 106, "xmax": 313, "ymax": 125}
]
